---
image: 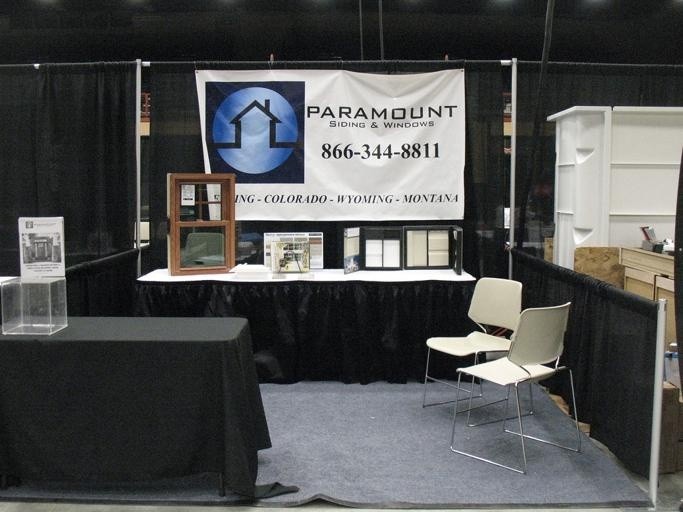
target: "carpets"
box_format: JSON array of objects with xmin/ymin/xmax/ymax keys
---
[{"xmin": 257, "ymin": 372, "xmax": 653, "ymax": 510}]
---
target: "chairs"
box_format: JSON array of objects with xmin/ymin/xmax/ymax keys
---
[{"xmin": 421, "ymin": 276, "xmax": 582, "ymax": 473}]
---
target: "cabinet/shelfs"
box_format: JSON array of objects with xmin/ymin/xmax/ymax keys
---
[{"xmin": 545, "ymin": 103, "xmax": 683, "ymax": 273}]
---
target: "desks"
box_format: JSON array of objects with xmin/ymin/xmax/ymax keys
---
[
  {"xmin": 134, "ymin": 265, "xmax": 477, "ymax": 385},
  {"xmin": 0, "ymin": 316, "xmax": 249, "ymax": 496}
]
